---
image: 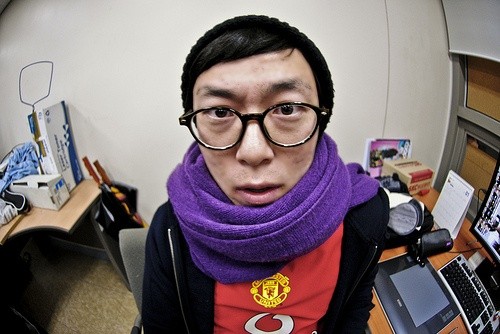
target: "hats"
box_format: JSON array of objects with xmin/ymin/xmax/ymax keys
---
[{"xmin": 180, "ymin": 14, "xmax": 334, "ymax": 116}]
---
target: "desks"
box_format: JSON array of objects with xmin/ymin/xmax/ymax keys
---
[
  {"xmin": 1, "ymin": 178, "xmax": 133, "ymax": 293},
  {"xmin": 365, "ymin": 183, "xmax": 496, "ymax": 334}
]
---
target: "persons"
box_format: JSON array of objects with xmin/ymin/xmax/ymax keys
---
[{"xmin": 140, "ymin": 15, "xmax": 390, "ymax": 334}]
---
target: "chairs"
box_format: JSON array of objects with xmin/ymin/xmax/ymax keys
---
[{"xmin": 119, "ymin": 229, "xmax": 150, "ymax": 334}]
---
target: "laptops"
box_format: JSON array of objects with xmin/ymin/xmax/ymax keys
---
[{"xmin": 371, "ymin": 251, "xmax": 461, "ymax": 334}]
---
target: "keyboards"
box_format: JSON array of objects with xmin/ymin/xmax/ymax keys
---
[{"xmin": 438, "ymin": 254, "xmax": 500, "ymax": 334}]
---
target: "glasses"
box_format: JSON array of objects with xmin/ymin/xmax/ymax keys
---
[{"xmin": 178, "ymin": 99, "xmax": 330, "ymax": 151}]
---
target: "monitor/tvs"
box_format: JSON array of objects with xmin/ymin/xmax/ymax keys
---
[{"xmin": 467, "ymin": 148, "xmax": 500, "ymax": 312}]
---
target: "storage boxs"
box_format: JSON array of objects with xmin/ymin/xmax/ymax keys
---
[
  {"xmin": 27, "ymin": 101, "xmax": 84, "ymax": 193},
  {"xmin": 382, "ymin": 159, "xmax": 434, "ymax": 196},
  {"xmin": 7, "ymin": 174, "xmax": 70, "ymax": 211}
]
